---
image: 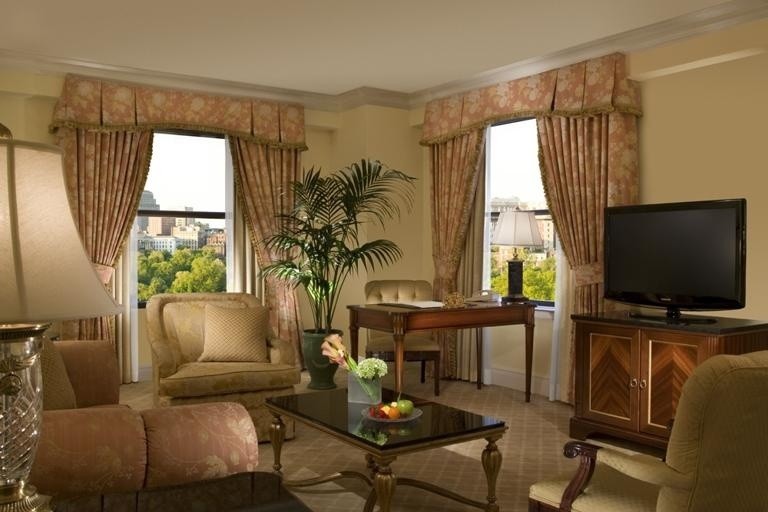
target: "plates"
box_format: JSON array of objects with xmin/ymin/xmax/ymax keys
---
[{"xmin": 361, "ymin": 406, "xmax": 422, "ymax": 424}]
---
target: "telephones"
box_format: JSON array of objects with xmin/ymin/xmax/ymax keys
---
[{"xmin": 466, "ymin": 290, "xmax": 502, "ymax": 302}]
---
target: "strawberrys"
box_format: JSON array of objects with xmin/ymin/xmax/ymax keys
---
[
  {"xmin": 378, "ymin": 403, "xmax": 384, "ymax": 408},
  {"xmin": 377, "ymin": 410, "xmax": 386, "ymax": 418},
  {"xmin": 370, "ymin": 407, "xmax": 376, "ymax": 416}
]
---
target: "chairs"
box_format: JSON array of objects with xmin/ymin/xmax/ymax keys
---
[
  {"xmin": 145, "ymin": 291, "xmax": 301, "ymax": 446},
  {"xmin": 527, "ymin": 349, "xmax": 767, "ymax": 508},
  {"xmin": 363, "ymin": 277, "xmax": 442, "ymax": 397}
]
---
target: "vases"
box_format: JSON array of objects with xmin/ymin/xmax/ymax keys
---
[{"xmin": 347, "ymin": 372, "xmax": 382, "ymax": 404}]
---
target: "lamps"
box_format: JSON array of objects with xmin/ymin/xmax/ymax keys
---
[
  {"xmin": 0, "ymin": 121, "xmax": 126, "ymax": 512},
  {"xmin": 486, "ymin": 209, "xmax": 545, "ymax": 306}
]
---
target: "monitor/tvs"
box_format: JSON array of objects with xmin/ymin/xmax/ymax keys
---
[{"xmin": 604, "ymin": 198, "xmax": 746, "ymax": 324}]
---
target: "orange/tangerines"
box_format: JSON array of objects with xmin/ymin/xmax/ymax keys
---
[{"xmin": 387, "ymin": 407, "xmax": 400, "ymax": 420}]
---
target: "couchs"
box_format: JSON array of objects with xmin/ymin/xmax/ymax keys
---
[{"xmin": 31, "ymin": 338, "xmax": 259, "ymax": 495}]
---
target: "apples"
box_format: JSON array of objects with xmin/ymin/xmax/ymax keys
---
[{"xmin": 397, "ymin": 400, "xmax": 414, "ymax": 418}]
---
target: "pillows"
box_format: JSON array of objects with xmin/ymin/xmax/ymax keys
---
[
  {"xmin": 40, "ymin": 334, "xmax": 79, "ymax": 409},
  {"xmin": 195, "ymin": 302, "xmax": 271, "ymax": 363}
]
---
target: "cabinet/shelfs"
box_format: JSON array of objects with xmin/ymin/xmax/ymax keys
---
[{"xmin": 565, "ymin": 307, "xmax": 767, "ymax": 453}]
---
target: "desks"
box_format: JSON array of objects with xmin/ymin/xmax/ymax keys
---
[
  {"xmin": 346, "ymin": 295, "xmax": 537, "ymax": 403},
  {"xmin": 49, "ymin": 471, "xmax": 314, "ymax": 511}
]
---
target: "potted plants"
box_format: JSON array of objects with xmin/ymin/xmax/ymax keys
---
[{"xmin": 253, "ymin": 156, "xmax": 418, "ymax": 392}]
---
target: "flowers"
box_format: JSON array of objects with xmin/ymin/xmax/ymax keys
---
[
  {"xmin": 355, "ymin": 419, "xmax": 391, "ymax": 446},
  {"xmin": 321, "ymin": 333, "xmax": 390, "ymax": 406}
]
